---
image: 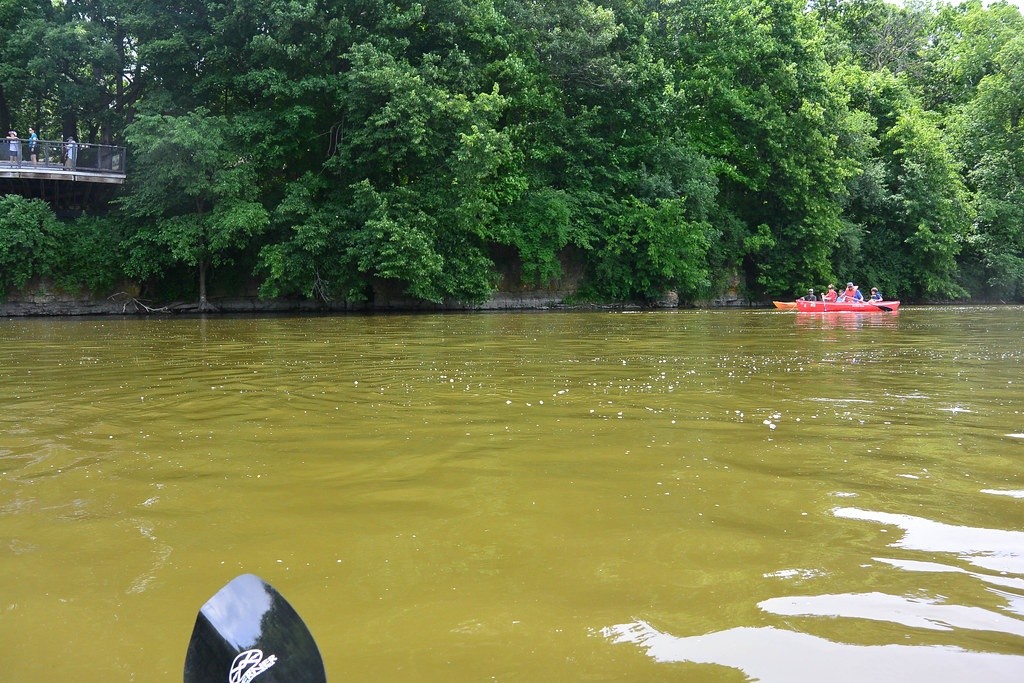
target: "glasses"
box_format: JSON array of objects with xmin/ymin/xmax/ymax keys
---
[{"xmin": 809, "ymin": 291, "xmax": 813, "ymax": 292}]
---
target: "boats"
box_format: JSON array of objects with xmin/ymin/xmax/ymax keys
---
[
  {"xmin": 794, "ymin": 298, "xmax": 901, "ymax": 312},
  {"xmin": 772, "ymin": 301, "xmax": 798, "ymax": 312}
]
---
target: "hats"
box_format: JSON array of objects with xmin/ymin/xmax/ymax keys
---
[
  {"xmin": 871, "ymin": 287, "xmax": 878, "ymax": 292},
  {"xmin": 8, "ymin": 131, "xmax": 18, "ymax": 137}
]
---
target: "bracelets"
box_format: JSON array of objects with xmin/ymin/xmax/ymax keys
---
[{"xmin": 32, "ymin": 147, "xmax": 35, "ymax": 148}]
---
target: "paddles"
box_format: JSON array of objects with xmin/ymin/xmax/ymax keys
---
[
  {"xmin": 843, "ymin": 294, "xmax": 893, "ymax": 312},
  {"xmin": 821, "ymin": 293, "xmax": 828, "ymax": 311}
]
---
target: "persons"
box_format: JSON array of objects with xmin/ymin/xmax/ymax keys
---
[
  {"xmin": 800, "ymin": 282, "xmax": 883, "ymax": 303},
  {"xmin": 29, "ymin": 128, "xmax": 37, "ymax": 165},
  {"xmin": 63, "ymin": 137, "xmax": 78, "ymax": 172},
  {"xmin": 6, "ymin": 131, "xmax": 19, "ymax": 165}
]
